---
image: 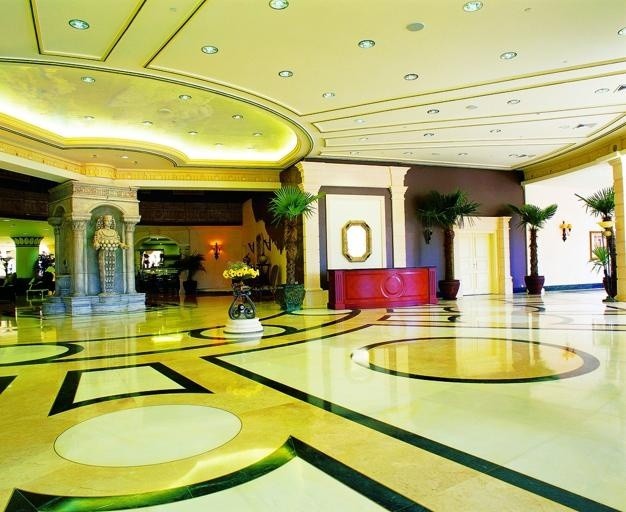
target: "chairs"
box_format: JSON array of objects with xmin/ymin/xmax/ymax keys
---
[{"xmin": 253, "ymin": 264, "xmax": 279, "ymax": 303}]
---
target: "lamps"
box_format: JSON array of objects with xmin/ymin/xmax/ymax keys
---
[{"xmin": 560, "ymin": 221, "xmax": 573, "ymax": 242}]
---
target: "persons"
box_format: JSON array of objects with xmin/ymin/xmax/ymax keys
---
[{"xmin": 89, "ymin": 214, "xmax": 128, "ymax": 252}]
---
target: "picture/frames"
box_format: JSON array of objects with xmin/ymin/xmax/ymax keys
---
[{"xmin": 589, "ymin": 230, "xmax": 606, "ymax": 261}]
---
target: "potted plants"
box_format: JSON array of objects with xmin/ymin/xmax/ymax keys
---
[
  {"xmin": 266, "ymin": 183, "xmax": 326, "ymax": 314},
  {"xmin": 414, "ymin": 187, "xmax": 483, "ymax": 300},
  {"xmin": 574, "ymin": 187, "xmax": 617, "ymax": 302},
  {"xmin": 173, "ymin": 254, "xmax": 208, "ymax": 295},
  {"xmin": 508, "ymin": 202, "xmax": 557, "ymax": 295}
]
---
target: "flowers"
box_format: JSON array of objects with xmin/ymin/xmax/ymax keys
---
[{"xmin": 221, "ymin": 261, "xmax": 260, "ymax": 281}]
